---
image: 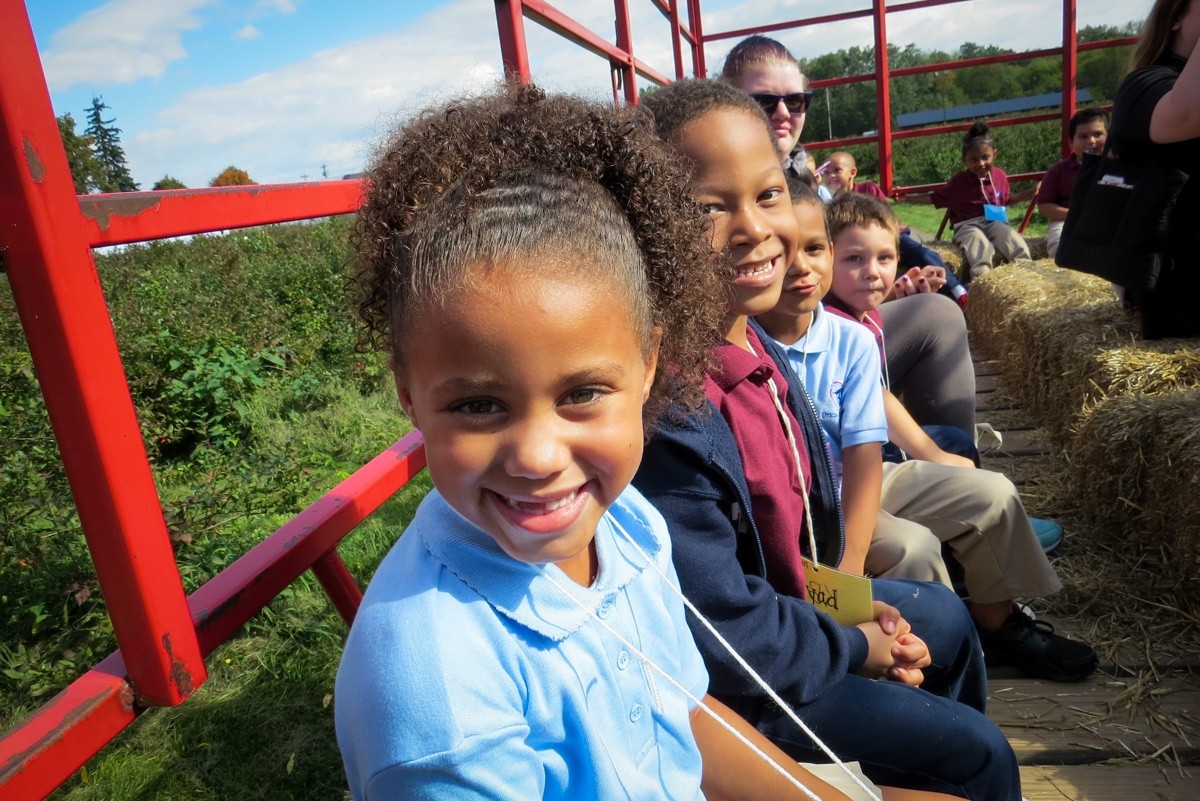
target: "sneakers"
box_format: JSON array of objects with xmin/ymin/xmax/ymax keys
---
[
  {"xmin": 984, "ymin": 599, "xmax": 1098, "ymax": 683},
  {"xmin": 1028, "ymin": 516, "xmax": 1064, "ymax": 554}
]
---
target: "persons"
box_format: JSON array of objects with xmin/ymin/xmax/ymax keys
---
[
  {"xmin": 750, "ymin": 173, "xmax": 1099, "ymax": 683},
  {"xmin": 626, "ymin": 79, "xmax": 1024, "ymax": 801},
  {"xmin": 824, "ymin": 191, "xmax": 1064, "ymax": 557},
  {"xmin": 332, "ymin": 88, "xmax": 969, "ymax": 801},
  {"xmin": 712, "ymin": 33, "xmax": 972, "ymax": 314},
  {"xmin": 1099, "ymin": 0, "xmax": 1200, "ymax": 345},
  {"xmin": 1038, "ymin": 108, "xmax": 1109, "ymax": 256},
  {"xmin": 930, "ymin": 123, "xmax": 1033, "ymax": 283}
]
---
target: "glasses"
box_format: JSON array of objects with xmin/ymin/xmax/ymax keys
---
[{"xmin": 749, "ymin": 91, "xmax": 814, "ymax": 114}]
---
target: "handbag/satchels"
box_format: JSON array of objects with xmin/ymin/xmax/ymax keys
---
[{"xmin": 1055, "ymin": 150, "xmax": 1189, "ymax": 293}]
